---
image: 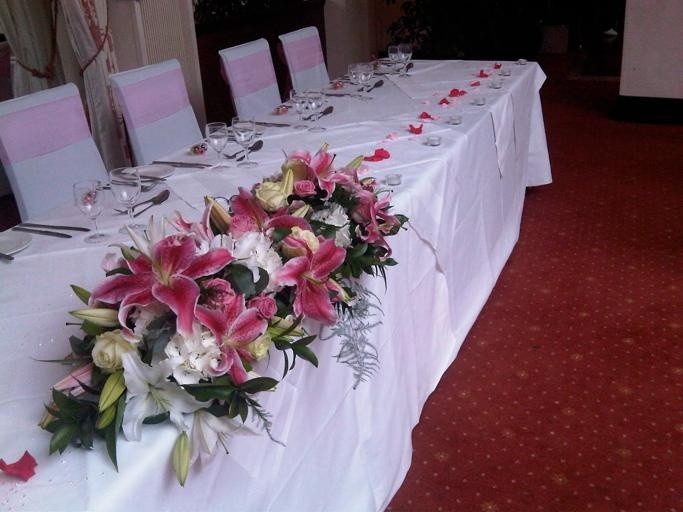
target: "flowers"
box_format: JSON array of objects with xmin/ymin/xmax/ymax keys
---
[{"xmin": 33, "ymin": 138, "xmax": 409, "ymax": 487}]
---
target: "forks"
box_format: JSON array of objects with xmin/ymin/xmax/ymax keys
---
[
  {"xmin": 114, "ymin": 194, "xmax": 168, "ymax": 215},
  {"xmin": 220, "ymin": 143, "xmax": 252, "ymax": 159}
]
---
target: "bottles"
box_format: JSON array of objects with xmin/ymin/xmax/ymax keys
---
[{"xmin": 518, "ymin": 59, "xmax": 527, "ymax": 65}]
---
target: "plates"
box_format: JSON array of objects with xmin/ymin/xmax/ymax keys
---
[
  {"xmin": 108, "ymin": 163, "xmax": 176, "ymax": 184},
  {"xmin": 167, "ymin": 153, "xmax": 221, "ymax": 174},
  {"xmin": 0, "ymin": 231, "xmax": 32, "ymax": 258}
]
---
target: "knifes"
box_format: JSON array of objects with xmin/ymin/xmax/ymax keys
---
[
  {"xmin": 0, "ymin": 251, "xmax": 13, "ymax": 260},
  {"xmin": 117, "ymin": 170, "xmax": 167, "ymax": 185},
  {"xmin": 245, "ymin": 116, "xmax": 290, "ymax": 129},
  {"xmin": 16, "ymin": 221, "xmax": 91, "ymax": 240}
]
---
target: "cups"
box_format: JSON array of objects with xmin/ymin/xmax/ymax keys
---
[
  {"xmin": 491, "ymin": 77, "xmax": 503, "ymax": 88},
  {"xmin": 473, "ymin": 98, "xmax": 485, "ymax": 107},
  {"xmin": 385, "ymin": 174, "xmax": 400, "ymax": 186},
  {"xmin": 499, "ymin": 66, "xmax": 509, "ymax": 76},
  {"xmin": 449, "ymin": 116, "xmax": 462, "ymax": 125},
  {"xmin": 427, "ymin": 136, "xmax": 441, "ymax": 146}
]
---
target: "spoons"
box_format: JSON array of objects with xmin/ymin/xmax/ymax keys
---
[
  {"xmin": 236, "ymin": 139, "xmax": 263, "ymax": 162},
  {"xmin": 365, "ymin": 80, "xmax": 385, "ymax": 93},
  {"xmin": 312, "ymin": 106, "xmax": 333, "ymax": 122},
  {"xmin": 133, "ymin": 190, "xmax": 169, "ymax": 219},
  {"xmin": 404, "ymin": 64, "xmax": 413, "ymax": 75}
]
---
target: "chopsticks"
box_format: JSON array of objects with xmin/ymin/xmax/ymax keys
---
[{"xmin": 151, "ymin": 160, "xmax": 210, "ymax": 171}]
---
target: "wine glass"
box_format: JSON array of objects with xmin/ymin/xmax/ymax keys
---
[
  {"xmin": 71, "ymin": 167, "xmax": 147, "ymax": 242},
  {"xmin": 205, "ymin": 116, "xmax": 257, "ymax": 172},
  {"xmin": 288, "ymin": 88, "xmax": 326, "ymax": 133},
  {"xmin": 346, "ymin": 62, "xmax": 374, "ymax": 103},
  {"xmin": 387, "ymin": 44, "xmax": 413, "ymax": 80}
]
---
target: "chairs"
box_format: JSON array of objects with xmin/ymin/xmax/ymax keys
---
[
  {"xmin": 0, "ymin": 82, "xmax": 109, "ymax": 223},
  {"xmin": 217, "ymin": 38, "xmax": 283, "ymax": 120},
  {"xmin": 280, "ymin": 27, "xmax": 332, "ymax": 94},
  {"xmin": 109, "ymin": 58, "xmax": 204, "ymax": 167}
]
